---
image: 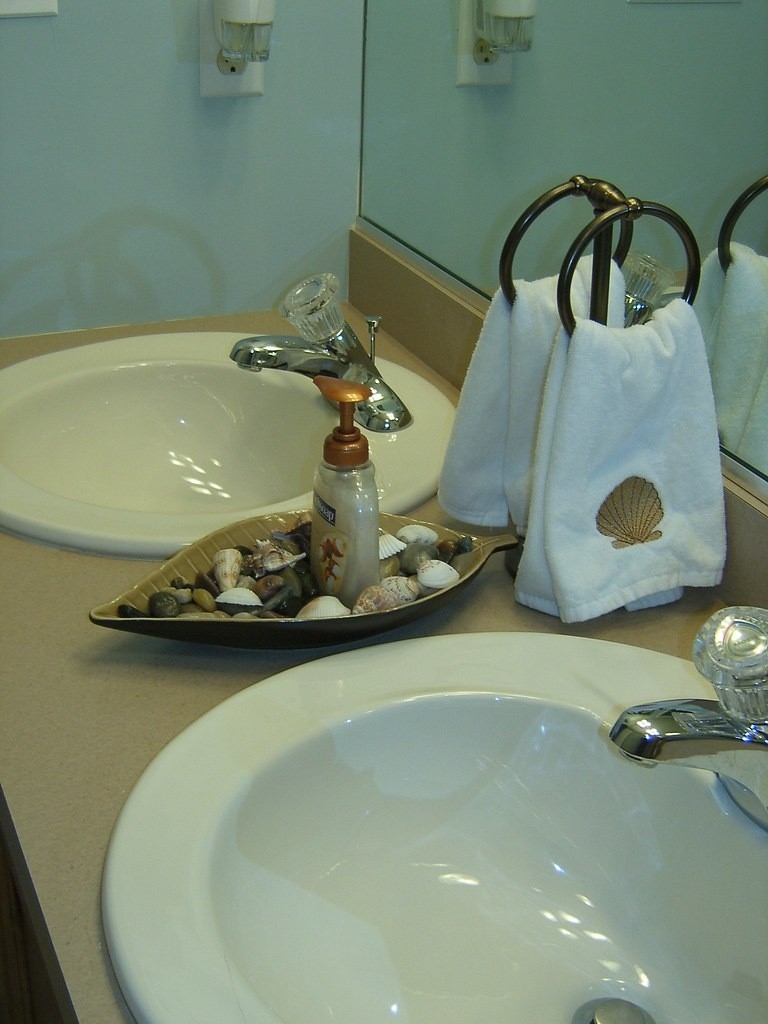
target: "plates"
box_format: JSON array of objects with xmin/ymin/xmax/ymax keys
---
[{"xmin": 86, "ymin": 508, "xmax": 521, "ymax": 652}]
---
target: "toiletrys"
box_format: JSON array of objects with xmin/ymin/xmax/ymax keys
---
[{"xmin": 312, "ymin": 372, "xmax": 385, "ymax": 604}]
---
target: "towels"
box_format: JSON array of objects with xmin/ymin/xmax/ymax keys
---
[
  {"xmin": 436, "ymin": 254, "xmax": 630, "ymax": 529},
  {"xmin": 686, "ymin": 242, "xmax": 767, "ymax": 481},
  {"xmin": 513, "ymin": 300, "xmax": 728, "ymax": 625}
]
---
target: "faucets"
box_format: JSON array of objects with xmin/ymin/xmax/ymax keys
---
[
  {"xmin": 606, "ymin": 604, "xmax": 768, "ymax": 845},
  {"xmin": 227, "ymin": 270, "xmax": 413, "ymax": 433}
]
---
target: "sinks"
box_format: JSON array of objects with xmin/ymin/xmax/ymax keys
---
[
  {"xmin": 1, "ymin": 331, "xmax": 465, "ymax": 563},
  {"xmin": 96, "ymin": 627, "xmax": 768, "ymax": 1024}
]
